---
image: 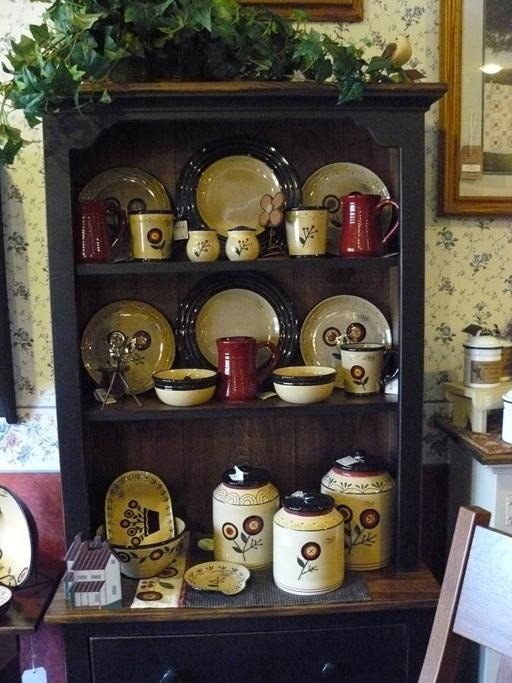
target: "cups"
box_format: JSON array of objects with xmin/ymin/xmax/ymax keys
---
[
  {"xmin": 338, "ymin": 192, "xmax": 399, "ymax": 258},
  {"xmin": 131, "ymin": 211, "xmax": 173, "ymax": 264},
  {"xmin": 73, "ymin": 202, "xmax": 126, "ymax": 264},
  {"xmin": 285, "ymin": 205, "xmax": 330, "ymax": 257},
  {"xmin": 216, "ymin": 336, "xmax": 281, "ymax": 405},
  {"xmin": 339, "ymin": 343, "xmax": 399, "ymax": 398}
]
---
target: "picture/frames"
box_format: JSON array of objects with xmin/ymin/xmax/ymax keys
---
[{"xmin": 439, "ymin": 1, "xmax": 512, "ymax": 217}]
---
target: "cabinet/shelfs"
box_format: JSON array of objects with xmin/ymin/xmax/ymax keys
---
[
  {"xmin": 42, "ymin": 78, "xmax": 451, "ymax": 682},
  {"xmin": 435, "ymin": 416, "xmax": 512, "ymax": 683}
]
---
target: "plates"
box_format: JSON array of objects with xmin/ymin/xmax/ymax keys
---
[
  {"xmin": 105, "ymin": 470, "xmax": 176, "ymax": 543},
  {"xmin": 185, "ymin": 561, "xmax": 250, "ymax": 596},
  {"xmin": 78, "ymin": 167, "xmax": 173, "ymax": 263},
  {"xmin": 80, "ymin": 299, "xmax": 176, "ymax": 394},
  {"xmin": 300, "ymin": 295, "xmax": 394, "ymax": 388},
  {"xmin": 174, "ymin": 272, "xmax": 300, "ymax": 390},
  {"xmin": 175, "ymin": 138, "xmax": 300, "ymax": 257},
  {"xmin": 301, "ymin": 162, "xmax": 392, "ymax": 256},
  {"xmin": 0, "ymin": 486, "xmax": 32, "ymax": 588},
  {"xmin": 0, "ymin": 586, "xmax": 13, "ymax": 608}
]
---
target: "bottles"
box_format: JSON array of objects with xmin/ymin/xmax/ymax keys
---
[
  {"xmin": 491, "ymin": 329, "xmax": 512, "ymax": 381},
  {"xmin": 462, "ymin": 327, "xmax": 502, "ymax": 388},
  {"xmin": 319, "ymin": 449, "xmax": 396, "ymax": 572},
  {"xmin": 272, "ymin": 491, "xmax": 345, "ymax": 595},
  {"xmin": 212, "ymin": 464, "xmax": 279, "ymax": 572},
  {"xmin": 502, "ymin": 389, "xmax": 512, "ymax": 444}
]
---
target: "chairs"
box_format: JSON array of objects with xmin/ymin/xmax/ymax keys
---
[{"xmin": 419, "ymin": 506, "xmax": 512, "ymax": 683}]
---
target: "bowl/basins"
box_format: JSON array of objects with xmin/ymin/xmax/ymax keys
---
[
  {"xmin": 152, "ymin": 367, "xmax": 216, "ymax": 407},
  {"xmin": 271, "ymin": 365, "xmax": 335, "ymax": 404},
  {"xmin": 96, "ymin": 517, "xmax": 184, "ymax": 580}
]
